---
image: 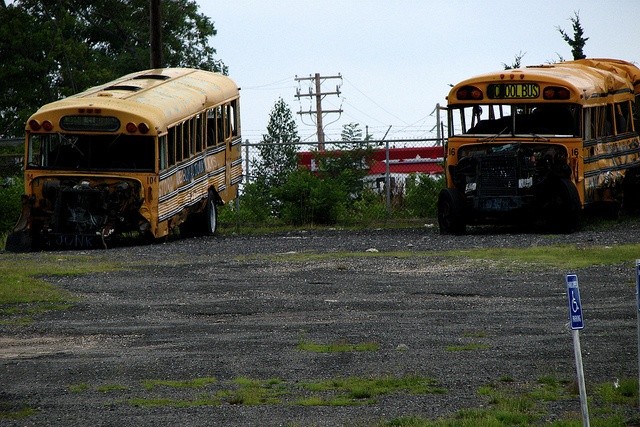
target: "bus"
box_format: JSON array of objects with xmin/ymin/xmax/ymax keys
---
[
  {"xmin": 7, "ymin": 67, "xmax": 242, "ymax": 250},
  {"xmin": 438, "ymin": 58, "xmax": 640, "ymax": 233}
]
